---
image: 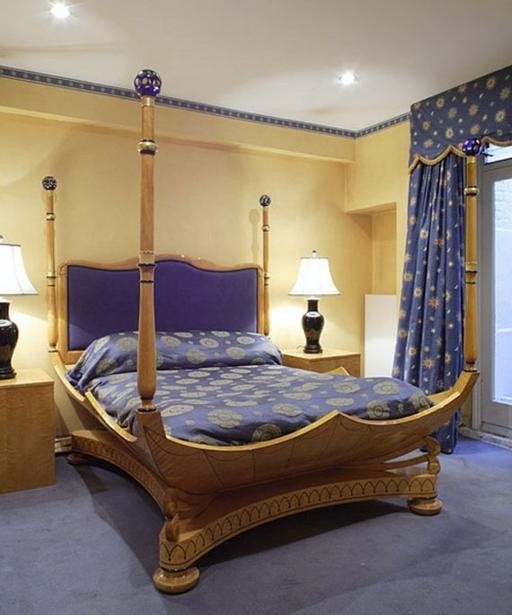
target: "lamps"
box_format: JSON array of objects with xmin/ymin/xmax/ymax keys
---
[
  {"xmin": 288, "ymin": 257, "xmax": 344, "ymax": 354},
  {"xmin": 0, "ymin": 241, "xmax": 40, "ymax": 381}
]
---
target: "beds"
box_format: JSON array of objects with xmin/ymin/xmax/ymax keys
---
[{"xmin": 46, "ymin": 251, "xmax": 493, "ymax": 595}]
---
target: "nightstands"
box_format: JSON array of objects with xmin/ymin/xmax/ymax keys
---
[
  {"xmin": 285, "ymin": 348, "xmax": 366, "ymax": 377},
  {"xmin": 0, "ymin": 368, "xmax": 54, "ymax": 494}
]
